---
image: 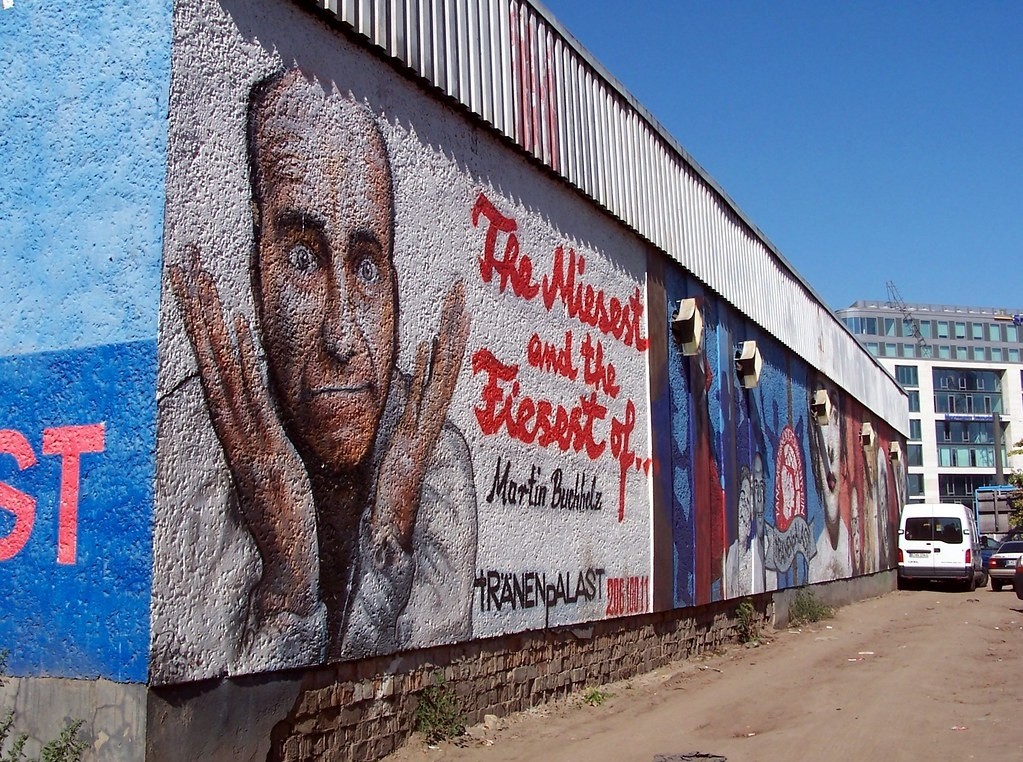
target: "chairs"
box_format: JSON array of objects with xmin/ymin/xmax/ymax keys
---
[{"xmin": 943, "ymin": 525, "xmax": 958, "ymax": 541}]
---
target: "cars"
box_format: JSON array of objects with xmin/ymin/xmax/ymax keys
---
[
  {"xmin": 980, "ymin": 537, "xmax": 1003, "ymax": 567},
  {"xmin": 988, "ymin": 541, "xmax": 1023, "ymax": 592},
  {"xmin": 1013, "ymin": 555, "xmax": 1023, "ymax": 600}
]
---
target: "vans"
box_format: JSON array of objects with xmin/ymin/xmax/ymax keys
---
[{"xmin": 897, "ymin": 502, "xmax": 989, "ymax": 592}]
